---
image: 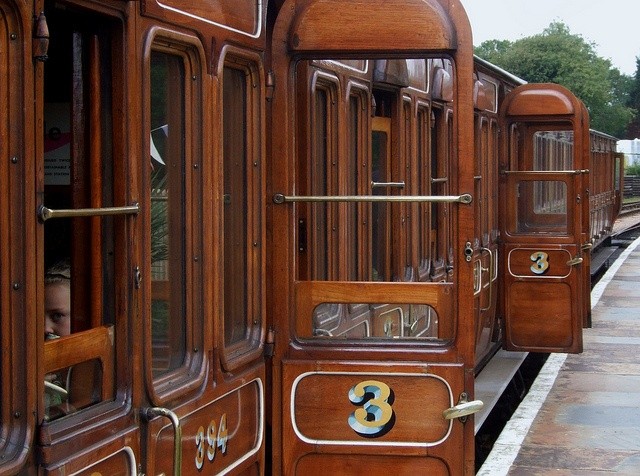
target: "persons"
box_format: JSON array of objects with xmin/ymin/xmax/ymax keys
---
[{"xmin": 41, "ymin": 258, "xmax": 99, "ymax": 421}]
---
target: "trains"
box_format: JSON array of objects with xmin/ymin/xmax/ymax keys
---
[{"xmin": 1, "ymin": 0, "xmax": 625, "ymax": 476}]
